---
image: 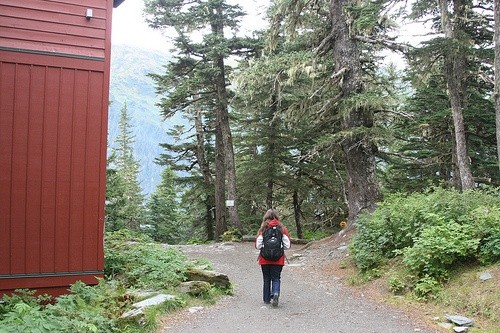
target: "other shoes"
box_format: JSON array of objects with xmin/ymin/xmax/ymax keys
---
[
  {"xmin": 264, "ymin": 301, "xmax": 270, "ymax": 303},
  {"xmin": 272, "ymin": 293, "xmax": 278, "ymax": 307}
]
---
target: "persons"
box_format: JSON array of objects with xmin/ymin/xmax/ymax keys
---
[{"xmin": 255, "ymin": 207, "xmax": 290, "ymax": 307}]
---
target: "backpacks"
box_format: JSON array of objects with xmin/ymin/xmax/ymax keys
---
[{"xmin": 260, "ymin": 225, "xmax": 284, "ymax": 260}]
